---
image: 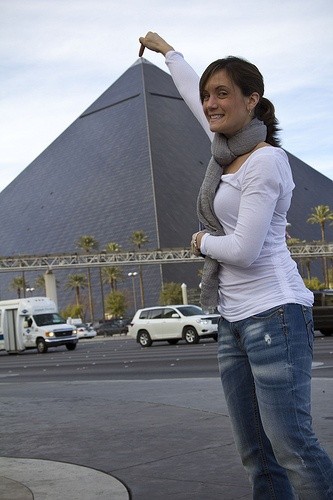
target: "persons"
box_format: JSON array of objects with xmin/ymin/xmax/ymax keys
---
[{"xmin": 138, "ymin": 31, "xmax": 332, "ymax": 500}]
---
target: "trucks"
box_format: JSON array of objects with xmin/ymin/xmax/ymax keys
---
[{"xmin": 0, "ymin": 297, "xmax": 79, "ymax": 354}]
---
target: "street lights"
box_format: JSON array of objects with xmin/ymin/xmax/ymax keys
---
[
  {"xmin": 25, "ymin": 287, "xmax": 35, "ymax": 298},
  {"xmin": 128, "ymin": 272, "xmax": 138, "ymax": 314}
]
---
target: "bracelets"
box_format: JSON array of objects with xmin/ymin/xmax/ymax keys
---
[{"xmin": 194, "ymin": 230, "xmax": 205, "ymax": 253}]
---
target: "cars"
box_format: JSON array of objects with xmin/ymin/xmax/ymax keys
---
[
  {"xmin": 75, "ymin": 324, "xmax": 97, "ymax": 339},
  {"xmin": 309, "ymin": 289, "xmax": 333, "ymax": 336},
  {"xmin": 127, "ymin": 304, "xmax": 222, "ymax": 347},
  {"xmin": 93, "ymin": 322, "xmax": 129, "ymax": 337}
]
